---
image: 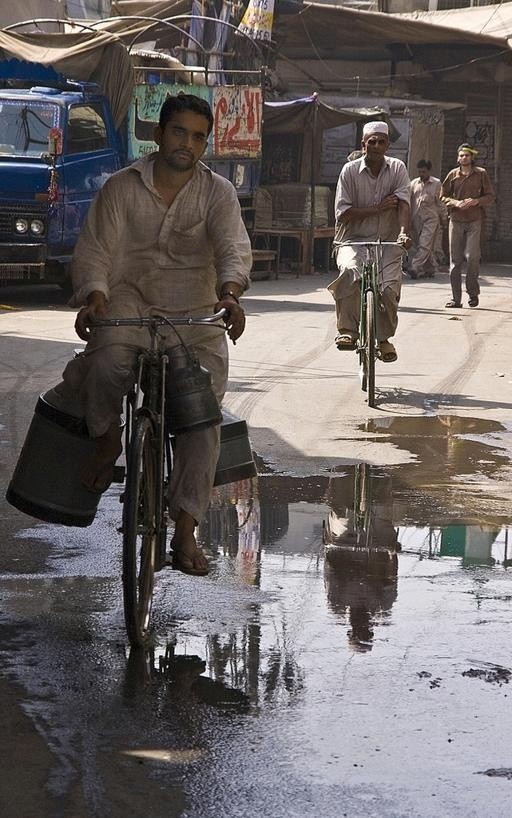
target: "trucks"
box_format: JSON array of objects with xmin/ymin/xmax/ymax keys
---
[{"xmin": 0, "ymin": 13, "xmax": 268, "ymax": 303}]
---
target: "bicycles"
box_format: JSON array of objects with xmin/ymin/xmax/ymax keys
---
[
  {"xmin": 321, "ymin": 460, "xmax": 401, "ymax": 563},
  {"xmin": 79, "ymin": 304, "xmax": 235, "ymax": 653},
  {"xmin": 329, "ymin": 236, "xmax": 411, "ymax": 409}
]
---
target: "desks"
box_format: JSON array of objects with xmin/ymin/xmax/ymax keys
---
[{"xmin": 251, "ymin": 227, "xmax": 335, "ymax": 275}]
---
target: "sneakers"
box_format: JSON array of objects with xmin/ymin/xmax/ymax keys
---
[
  {"xmin": 424, "ymin": 272, "xmax": 435, "ymax": 278},
  {"xmin": 407, "ymin": 268, "xmax": 418, "ymax": 279}
]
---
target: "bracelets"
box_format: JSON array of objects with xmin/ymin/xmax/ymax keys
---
[{"xmin": 217, "ymin": 291, "xmax": 241, "ymax": 304}]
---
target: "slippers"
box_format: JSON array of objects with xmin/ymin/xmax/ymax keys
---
[
  {"xmin": 378, "ymin": 343, "xmax": 397, "ymax": 362},
  {"xmin": 82, "ymin": 445, "xmax": 122, "ymax": 494},
  {"xmin": 468, "ymin": 296, "xmax": 478, "ymax": 307},
  {"xmin": 445, "ymin": 301, "xmax": 463, "ymax": 308},
  {"xmin": 171, "ymin": 538, "xmax": 210, "ymax": 576},
  {"xmin": 335, "ymin": 330, "xmax": 360, "ymax": 346}
]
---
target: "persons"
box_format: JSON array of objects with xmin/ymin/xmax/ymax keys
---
[
  {"xmin": 401, "ymin": 160, "xmax": 449, "ymax": 277},
  {"xmin": 326, "ymin": 512, "xmax": 400, "ymax": 655},
  {"xmin": 68, "ymin": 95, "xmax": 256, "ymax": 578},
  {"xmin": 441, "ymin": 142, "xmax": 495, "ymax": 309},
  {"xmin": 325, "ymin": 120, "xmax": 414, "ymax": 364}
]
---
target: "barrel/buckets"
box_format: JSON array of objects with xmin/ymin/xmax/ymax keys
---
[
  {"xmin": 165, "ymin": 343, "xmax": 223, "ymax": 434},
  {"xmin": 7, "ymin": 349, "xmax": 125, "ymax": 527},
  {"xmin": 212, "ymin": 419, "xmax": 257, "ymax": 488}
]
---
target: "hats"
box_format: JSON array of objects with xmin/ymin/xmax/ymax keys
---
[
  {"xmin": 457, "ymin": 143, "xmax": 478, "ymax": 154},
  {"xmin": 363, "ymin": 121, "xmax": 389, "ymax": 142}
]
